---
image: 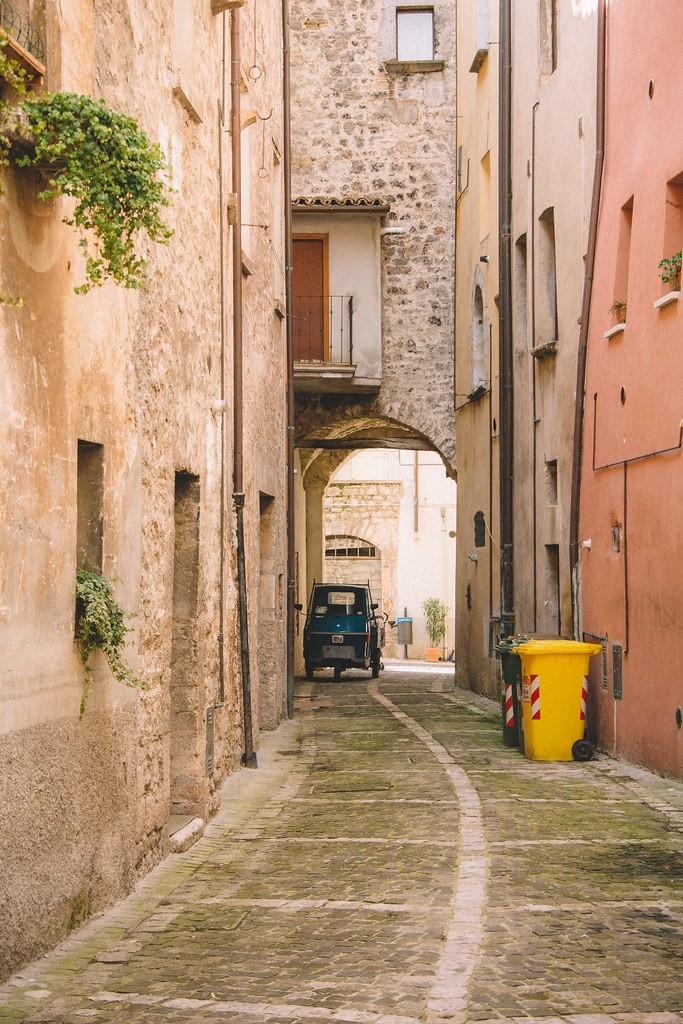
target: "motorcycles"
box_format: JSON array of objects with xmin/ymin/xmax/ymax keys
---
[{"xmin": 294, "ymin": 578, "xmax": 386, "ymax": 682}]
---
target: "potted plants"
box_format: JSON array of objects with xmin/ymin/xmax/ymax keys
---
[
  {"xmin": 1, "ymin": 34, "xmax": 175, "ymax": 293},
  {"xmin": 608, "ymin": 298, "xmax": 627, "ymax": 323},
  {"xmin": 68, "ymin": 559, "xmax": 153, "ymax": 720},
  {"xmin": 423, "ymin": 596, "xmax": 452, "ymax": 661},
  {"xmin": 657, "ymin": 251, "xmax": 683, "ymax": 292}
]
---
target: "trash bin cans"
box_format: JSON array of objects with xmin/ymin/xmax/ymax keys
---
[
  {"xmin": 397, "ymin": 617, "xmax": 413, "ymax": 644},
  {"xmin": 494, "ymin": 636, "xmax": 603, "ymax": 762}
]
